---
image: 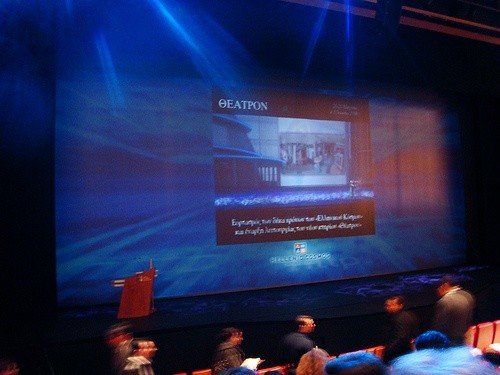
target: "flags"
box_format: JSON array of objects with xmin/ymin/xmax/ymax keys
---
[{"xmin": 118, "ymin": 268, "xmax": 155, "ymax": 318}]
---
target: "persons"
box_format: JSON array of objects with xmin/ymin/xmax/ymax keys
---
[
  {"xmin": 122, "ymin": 339, "xmax": 159, "ymax": 375},
  {"xmin": 295, "ymin": 347, "xmax": 330, "ymax": 375},
  {"xmin": 0, "ymin": 358, "xmax": 20, "ymax": 375},
  {"xmin": 323, "ymin": 352, "xmax": 388, "ymax": 375},
  {"xmin": 279, "ymin": 142, "xmax": 342, "ymax": 174},
  {"xmin": 264, "ymin": 370, "xmax": 283, "ymax": 375},
  {"xmin": 210, "ymin": 327, "xmax": 244, "ymax": 375},
  {"xmin": 386, "ymin": 344, "xmax": 500, "ymax": 375},
  {"xmin": 414, "ymin": 331, "xmax": 451, "ymax": 353},
  {"xmin": 433, "ymin": 275, "xmax": 476, "ymax": 346},
  {"xmin": 383, "ymin": 294, "xmax": 417, "ymax": 361},
  {"xmin": 219, "ymin": 367, "xmax": 259, "ymax": 375},
  {"xmin": 108, "ymin": 323, "xmax": 136, "ymax": 375},
  {"xmin": 283, "ymin": 315, "xmax": 316, "ymax": 375}
]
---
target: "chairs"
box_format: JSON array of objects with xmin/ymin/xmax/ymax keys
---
[
  {"xmin": 465, "ymin": 320, "xmax": 500, "ymax": 350},
  {"xmin": 255, "ymin": 346, "xmax": 385, "ymax": 375},
  {"xmin": 176, "ymin": 369, "xmax": 212, "ymax": 375}
]
